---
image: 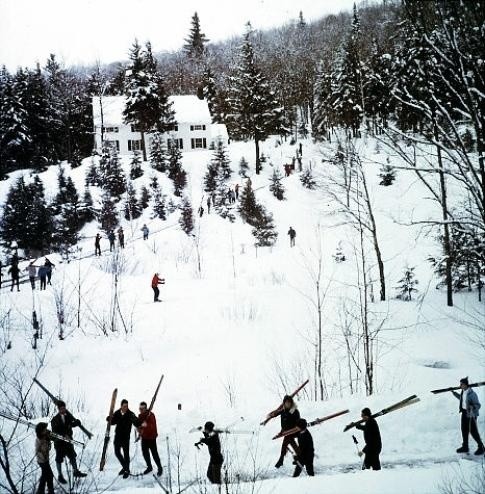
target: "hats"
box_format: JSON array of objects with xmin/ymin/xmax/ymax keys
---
[{"xmin": 460, "ymin": 377, "xmax": 468, "ymax": 385}]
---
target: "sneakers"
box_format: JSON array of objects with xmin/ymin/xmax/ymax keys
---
[
  {"xmin": 73, "ymin": 471, "xmax": 87, "ymax": 477},
  {"xmin": 144, "ymin": 468, "xmax": 152, "ymax": 474},
  {"xmin": 119, "ymin": 468, "xmax": 130, "ymax": 478},
  {"xmin": 474, "ymin": 445, "xmax": 485, "ymax": 454},
  {"xmin": 59, "ymin": 474, "xmax": 67, "ymax": 484},
  {"xmin": 157, "ymin": 469, "xmax": 162, "ymax": 476},
  {"xmin": 457, "ymin": 447, "xmax": 469, "ymax": 452}
]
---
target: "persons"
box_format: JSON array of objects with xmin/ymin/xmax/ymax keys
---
[
  {"xmin": 106, "ymin": 399, "xmax": 148, "ymax": 478},
  {"xmin": 450, "ymin": 377, "xmax": 485, "ymax": 455},
  {"xmin": 95, "ymin": 224, "xmax": 124, "ymax": 256},
  {"xmin": 136, "ymin": 402, "xmax": 162, "ymax": 477},
  {"xmin": 140, "ymin": 224, "xmax": 149, "ymax": 240},
  {"xmin": 355, "ymin": 408, "xmax": 382, "ymax": 470},
  {"xmin": 268, "ymin": 395, "xmax": 300, "ymax": 468},
  {"xmin": 7, "ymin": 253, "xmax": 54, "ymax": 292},
  {"xmin": 50, "ymin": 401, "xmax": 87, "ymax": 484},
  {"xmin": 288, "ymin": 226, "xmax": 295, "ymax": 247},
  {"xmin": 151, "ymin": 273, "xmax": 165, "ymax": 302},
  {"xmin": 198, "ymin": 184, "xmax": 242, "ymax": 217},
  {"xmin": 278, "ymin": 418, "xmax": 314, "ymax": 477},
  {"xmin": 199, "ymin": 422, "xmax": 223, "ymax": 484},
  {"xmin": 35, "ymin": 422, "xmax": 54, "ymax": 494}
]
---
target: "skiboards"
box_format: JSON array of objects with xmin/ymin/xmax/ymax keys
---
[
  {"xmin": 100, "ymin": 388, "xmax": 117, "ymax": 470},
  {"xmin": 33, "ymin": 377, "xmax": 93, "ymax": 440},
  {"xmin": 0, "ymin": 412, "xmax": 83, "ymax": 447},
  {"xmin": 188, "ymin": 417, "xmax": 257, "ymax": 449},
  {"xmin": 260, "ymin": 380, "xmax": 309, "ymax": 425},
  {"xmin": 343, "ymin": 395, "xmax": 421, "ymax": 432},
  {"xmin": 272, "ymin": 410, "xmax": 349, "ymax": 440},
  {"xmin": 134, "ymin": 374, "xmax": 165, "ymax": 443},
  {"xmin": 430, "ymin": 381, "xmax": 485, "ymax": 394}
]
